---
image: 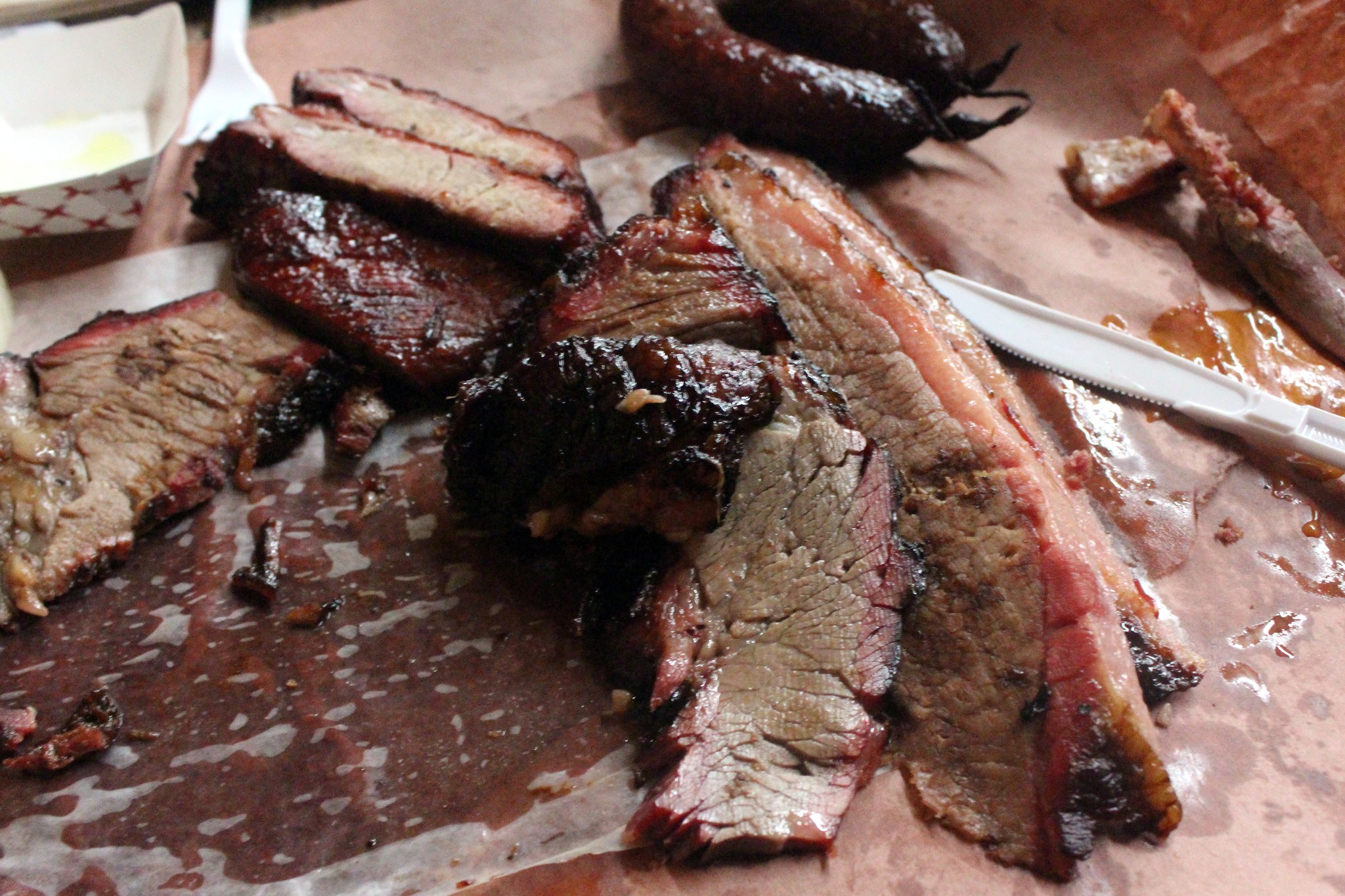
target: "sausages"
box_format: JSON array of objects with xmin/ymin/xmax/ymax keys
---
[{"xmin": 620, "ymin": 0, "xmax": 975, "ymax": 165}]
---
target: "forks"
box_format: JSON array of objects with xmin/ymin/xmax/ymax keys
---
[{"xmin": 178, "ymin": 0, "xmax": 275, "ymax": 147}]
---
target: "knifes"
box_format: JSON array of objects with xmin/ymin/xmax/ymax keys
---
[{"xmin": 923, "ymin": 269, "xmax": 1345, "ymax": 467}]
